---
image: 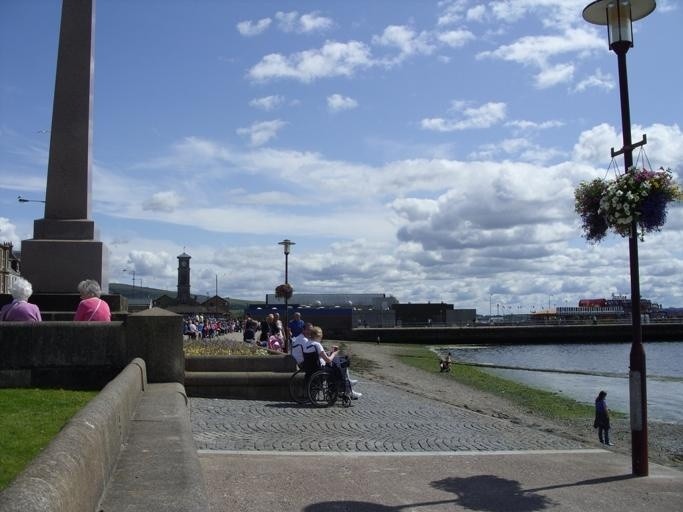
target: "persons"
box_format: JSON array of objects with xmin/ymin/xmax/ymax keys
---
[
  {"xmin": 439, "ymin": 360, "xmax": 444, "ymax": 372},
  {"xmin": 0, "ymin": 277, "xmax": 44, "ymax": 322},
  {"xmin": 182, "ymin": 309, "xmax": 363, "ymax": 401},
  {"xmin": 591, "ymin": 390, "xmax": 616, "ymax": 448},
  {"xmin": 69, "ymin": 278, "xmax": 113, "ymax": 323},
  {"xmin": 443, "ymin": 363, "xmax": 451, "ymax": 374},
  {"xmin": 444, "ymin": 352, "xmax": 452, "ymax": 364}
]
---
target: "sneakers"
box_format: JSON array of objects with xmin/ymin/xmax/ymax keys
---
[{"xmin": 347, "ymin": 378, "xmax": 362, "ymax": 400}]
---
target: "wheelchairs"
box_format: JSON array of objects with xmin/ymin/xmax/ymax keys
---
[{"xmin": 290, "ymin": 344, "xmax": 351, "ymax": 408}]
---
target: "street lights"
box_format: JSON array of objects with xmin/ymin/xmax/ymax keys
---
[
  {"xmin": 582, "ymin": 0, "xmax": 659, "ymax": 476},
  {"xmin": 123, "ymin": 267, "xmax": 148, "ymax": 300},
  {"xmin": 18, "ymin": 198, "xmax": 46, "ymax": 202},
  {"xmin": 278, "ymin": 238, "xmax": 296, "ymax": 354}
]
